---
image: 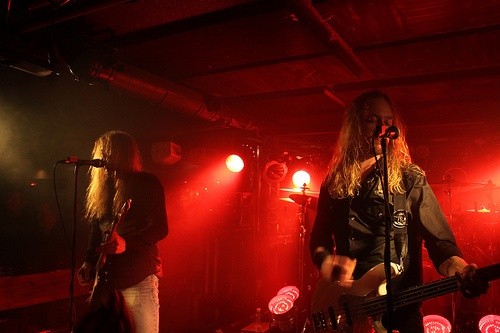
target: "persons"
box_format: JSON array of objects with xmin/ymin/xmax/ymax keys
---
[
  {"xmin": 78, "ymin": 131, "xmax": 168, "ymax": 333},
  {"xmin": 310, "ymin": 89, "xmax": 491, "ymax": 333},
  {"xmin": 1, "ymin": 190, "xmax": 75, "ymax": 276}
]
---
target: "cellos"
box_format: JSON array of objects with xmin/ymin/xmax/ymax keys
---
[{"xmin": 70, "ymin": 198, "xmax": 134, "ymax": 331}]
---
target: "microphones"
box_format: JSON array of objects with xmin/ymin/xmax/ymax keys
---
[
  {"xmin": 59, "ymin": 160, "xmax": 104, "ymax": 167},
  {"xmin": 383, "ymin": 126, "xmax": 399, "ymax": 138}
]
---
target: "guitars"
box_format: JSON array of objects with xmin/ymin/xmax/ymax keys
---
[{"xmin": 296, "ymin": 261, "xmax": 500, "ymax": 332}]
